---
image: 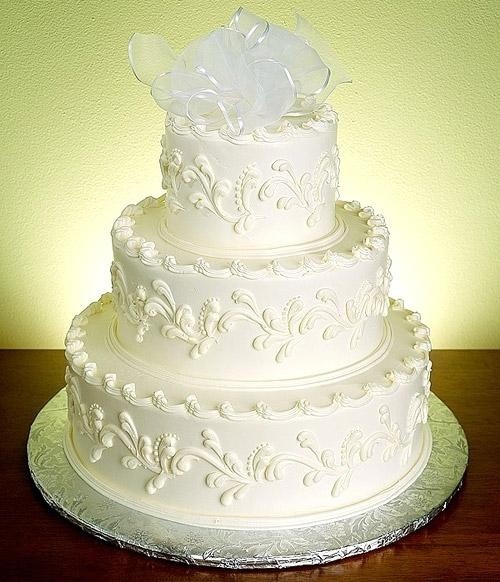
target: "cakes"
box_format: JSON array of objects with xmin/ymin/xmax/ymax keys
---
[{"xmin": 62, "ymin": 5, "xmax": 433, "ymax": 532}]
